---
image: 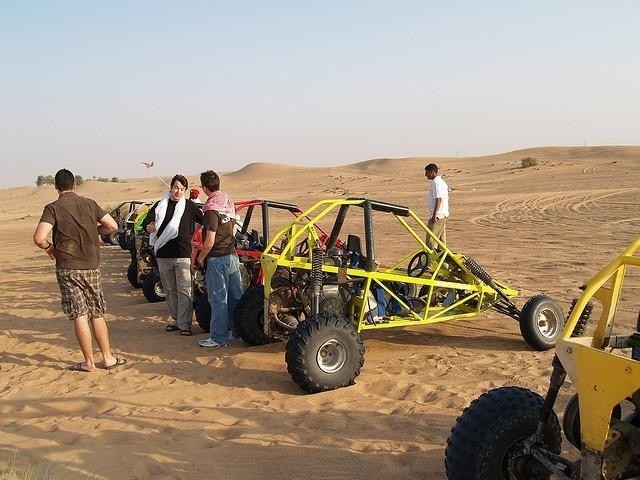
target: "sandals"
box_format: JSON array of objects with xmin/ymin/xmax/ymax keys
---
[
  {"xmin": 180, "ymin": 324, "xmax": 192, "ymax": 336},
  {"xmin": 166, "ymin": 325, "xmax": 179, "ymax": 331}
]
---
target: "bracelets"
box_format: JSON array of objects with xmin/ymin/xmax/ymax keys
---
[{"xmin": 45, "ymin": 244, "xmax": 53, "ymax": 250}]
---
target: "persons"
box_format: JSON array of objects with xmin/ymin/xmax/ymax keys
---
[
  {"xmin": 194, "ymin": 171, "xmax": 248, "ymax": 348},
  {"xmin": 187, "ymin": 188, "xmax": 203, "ymax": 213},
  {"xmin": 421, "ymin": 161, "xmax": 450, "ymax": 252},
  {"xmin": 35, "ymin": 170, "xmax": 127, "ymax": 373},
  {"xmin": 143, "ymin": 175, "xmax": 203, "ymax": 336}
]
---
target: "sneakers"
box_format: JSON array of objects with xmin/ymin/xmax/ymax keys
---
[{"xmin": 198, "ymin": 331, "xmax": 233, "ymax": 347}]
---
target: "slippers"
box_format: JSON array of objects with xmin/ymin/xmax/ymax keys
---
[
  {"xmin": 67, "ymin": 363, "xmax": 92, "ymax": 372},
  {"xmin": 104, "ymin": 358, "xmax": 127, "ymax": 370}
]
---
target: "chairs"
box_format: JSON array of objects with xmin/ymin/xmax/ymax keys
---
[
  {"xmin": 251, "ymin": 230, "xmax": 263, "ymax": 244},
  {"xmin": 346, "ymin": 235, "xmax": 379, "ymax": 270}
]
